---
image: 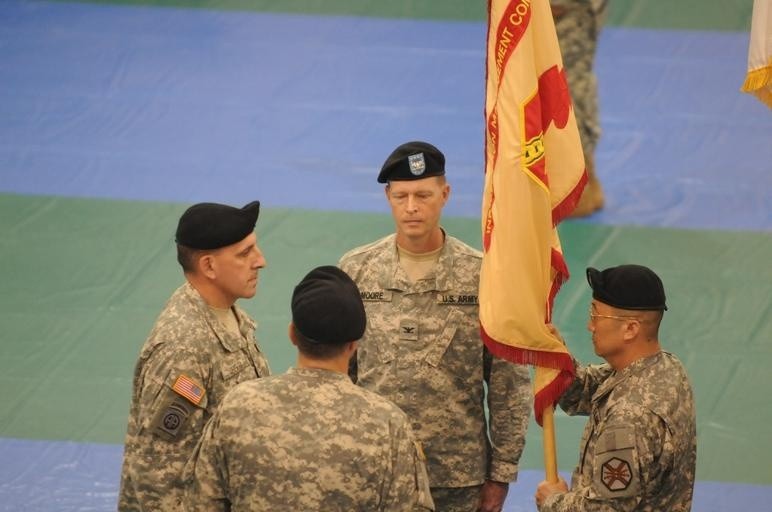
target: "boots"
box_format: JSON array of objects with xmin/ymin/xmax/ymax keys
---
[{"xmin": 566, "ymin": 160, "xmax": 604, "ymax": 217}]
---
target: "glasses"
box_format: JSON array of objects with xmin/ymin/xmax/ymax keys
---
[{"xmin": 589, "ymin": 305, "xmax": 636, "ymax": 320}]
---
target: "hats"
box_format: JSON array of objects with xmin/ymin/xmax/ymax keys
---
[
  {"xmin": 586, "ymin": 264, "xmax": 668, "ymax": 311},
  {"xmin": 377, "ymin": 141, "xmax": 445, "ymax": 183},
  {"xmin": 291, "ymin": 265, "xmax": 366, "ymax": 344},
  {"xmin": 175, "ymin": 200, "xmax": 260, "ymax": 248}
]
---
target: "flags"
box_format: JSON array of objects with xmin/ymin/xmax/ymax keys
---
[{"xmin": 478, "ymin": 0, "xmax": 588, "ymax": 427}]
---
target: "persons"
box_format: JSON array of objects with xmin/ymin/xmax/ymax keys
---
[
  {"xmin": 182, "ymin": 265, "xmax": 435, "ymax": 512},
  {"xmin": 336, "ymin": 141, "xmax": 532, "ymax": 512},
  {"xmin": 535, "ymin": 264, "xmax": 697, "ymax": 512},
  {"xmin": 117, "ymin": 200, "xmax": 271, "ymax": 512},
  {"xmin": 550, "ymin": 0, "xmax": 608, "ymax": 217}
]
